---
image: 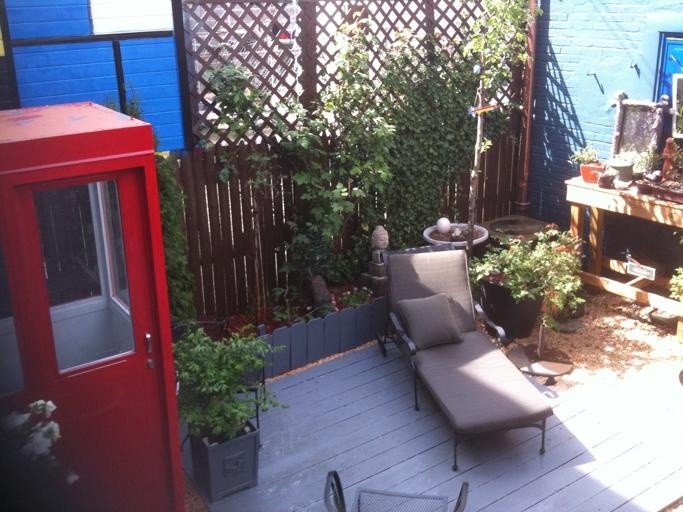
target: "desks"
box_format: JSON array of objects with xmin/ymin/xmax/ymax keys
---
[{"xmin": 564, "ymin": 174, "xmax": 683, "ymax": 316}]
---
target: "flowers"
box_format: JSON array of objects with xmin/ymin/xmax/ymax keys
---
[{"xmin": 467, "ymin": 224, "xmax": 587, "ymax": 329}]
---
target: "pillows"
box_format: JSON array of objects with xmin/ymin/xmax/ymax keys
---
[{"xmin": 392, "ymin": 291, "xmax": 464, "ymax": 352}]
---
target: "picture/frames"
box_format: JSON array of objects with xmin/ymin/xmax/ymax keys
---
[{"xmin": 608, "ymin": 30, "xmax": 682, "ymax": 178}]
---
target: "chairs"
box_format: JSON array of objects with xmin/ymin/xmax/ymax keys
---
[{"xmin": 372, "ymin": 249, "xmax": 554, "ymax": 473}]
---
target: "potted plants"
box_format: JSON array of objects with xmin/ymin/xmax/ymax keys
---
[
  {"xmin": 171, "ymin": 322, "xmax": 290, "ymax": 504},
  {"xmin": 568, "ymin": 148, "xmax": 606, "ymax": 184}
]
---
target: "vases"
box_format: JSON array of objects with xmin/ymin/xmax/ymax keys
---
[
  {"xmin": 422, "ymin": 223, "xmax": 488, "ymax": 258},
  {"xmin": 479, "ymin": 276, "xmax": 544, "ymax": 339}
]
